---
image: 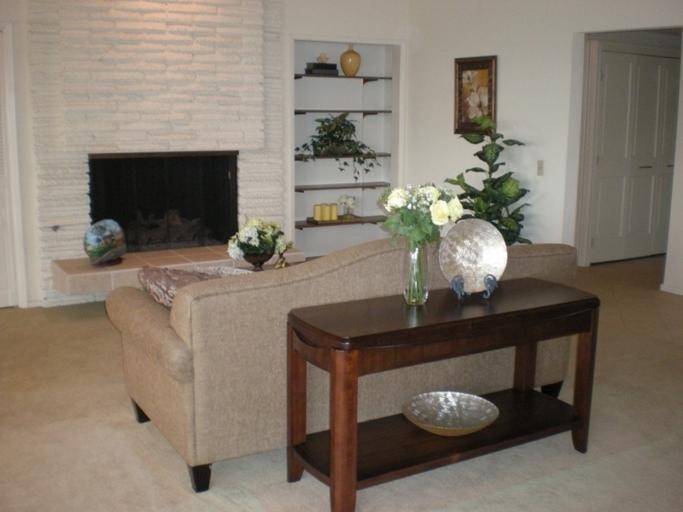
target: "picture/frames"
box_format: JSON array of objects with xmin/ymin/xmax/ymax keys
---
[{"xmin": 452, "ymin": 53, "xmax": 498, "ymax": 136}]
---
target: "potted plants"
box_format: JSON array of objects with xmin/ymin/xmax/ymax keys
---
[
  {"xmin": 293, "ymin": 107, "xmax": 383, "ymax": 182},
  {"xmin": 444, "ymin": 118, "xmax": 534, "ymax": 250}
]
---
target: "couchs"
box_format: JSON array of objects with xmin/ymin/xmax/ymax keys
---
[{"xmin": 104, "ymin": 232, "xmax": 578, "ymax": 494}]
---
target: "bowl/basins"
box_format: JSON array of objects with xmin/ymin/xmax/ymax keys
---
[{"xmin": 403, "ymin": 391, "xmax": 500, "ymax": 437}]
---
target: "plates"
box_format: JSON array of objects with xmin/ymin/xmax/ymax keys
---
[{"xmin": 437, "ymin": 217, "xmax": 509, "ymax": 296}]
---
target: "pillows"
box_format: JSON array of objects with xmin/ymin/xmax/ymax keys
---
[{"xmin": 139, "ymin": 262, "xmax": 218, "ymax": 310}]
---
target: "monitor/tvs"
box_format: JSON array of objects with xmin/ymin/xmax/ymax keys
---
[{"xmin": 87, "ymin": 150, "xmax": 239, "ymax": 254}]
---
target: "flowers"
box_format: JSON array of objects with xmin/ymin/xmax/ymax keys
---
[
  {"xmin": 226, "ymin": 215, "xmax": 294, "ymax": 261},
  {"xmin": 376, "ymin": 181, "xmax": 464, "ymax": 300}
]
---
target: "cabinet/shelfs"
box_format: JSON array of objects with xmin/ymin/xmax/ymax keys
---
[{"xmin": 293, "ymin": 41, "xmax": 395, "ymax": 231}]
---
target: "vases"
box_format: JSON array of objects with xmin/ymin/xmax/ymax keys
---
[
  {"xmin": 341, "ymin": 42, "xmax": 361, "ymax": 77},
  {"xmin": 399, "ymin": 236, "xmax": 430, "ymax": 307},
  {"xmin": 240, "ymin": 249, "xmax": 273, "ymax": 272}
]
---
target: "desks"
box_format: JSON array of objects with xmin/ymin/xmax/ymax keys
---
[{"xmin": 287, "ymin": 277, "xmax": 602, "ymax": 511}]
